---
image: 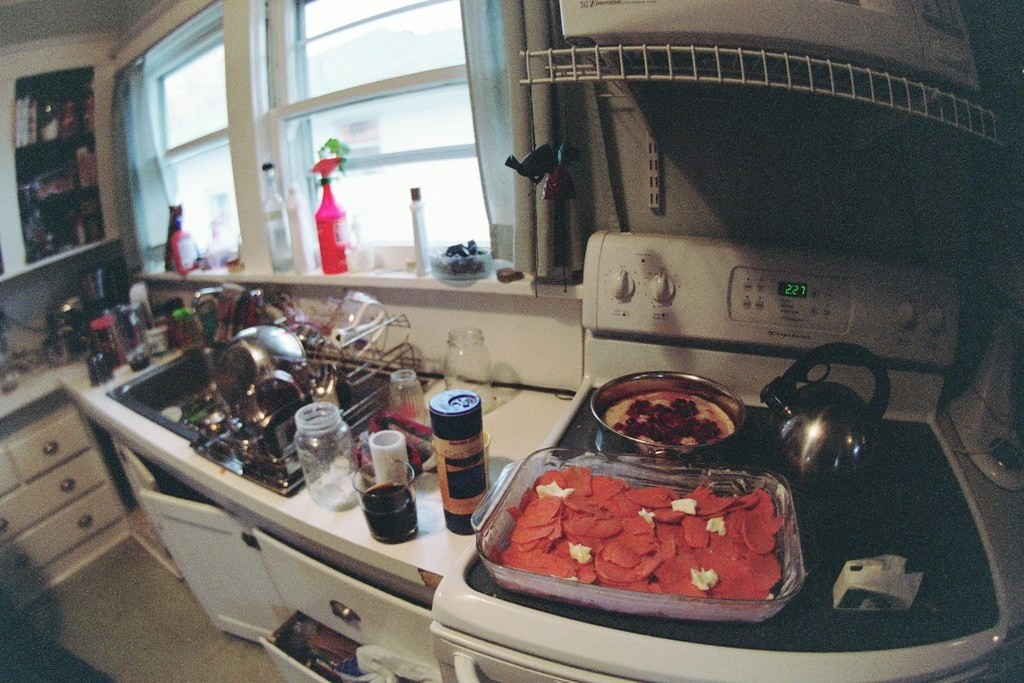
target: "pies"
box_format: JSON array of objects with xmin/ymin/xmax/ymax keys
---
[{"xmin": 600, "ymin": 390, "xmax": 735, "ymax": 446}]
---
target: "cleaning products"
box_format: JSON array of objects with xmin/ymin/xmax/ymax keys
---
[
  {"xmin": 285, "ymin": 156, "xmax": 348, "ymax": 276},
  {"xmin": 171, "ymin": 216, "xmax": 199, "ymax": 274}
]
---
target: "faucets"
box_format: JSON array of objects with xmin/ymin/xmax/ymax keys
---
[{"xmin": 192, "ymin": 282, "xmax": 246, "ymax": 341}]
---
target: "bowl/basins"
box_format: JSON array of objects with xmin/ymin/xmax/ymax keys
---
[
  {"xmin": 590, "ymin": 370, "xmax": 747, "ymax": 467},
  {"xmin": 467, "ymin": 446, "xmax": 808, "ymax": 624}
]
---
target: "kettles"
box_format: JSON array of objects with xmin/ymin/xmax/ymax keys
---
[{"xmin": 758, "ymin": 341, "xmax": 891, "ymax": 482}]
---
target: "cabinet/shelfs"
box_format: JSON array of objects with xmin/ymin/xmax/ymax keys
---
[
  {"xmin": 1, "ymin": 40, "xmax": 123, "ymax": 286},
  {"xmin": 0, "ymin": 402, "xmax": 444, "ymax": 683}
]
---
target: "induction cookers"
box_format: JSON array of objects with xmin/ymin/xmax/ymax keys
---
[{"xmin": 435, "ymin": 378, "xmax": 1006, "ymax": 683}]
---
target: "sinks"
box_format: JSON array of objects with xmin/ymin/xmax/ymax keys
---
[{"xmin": 104, "ymin": 338, "xmax": 448, "ymax": 496}]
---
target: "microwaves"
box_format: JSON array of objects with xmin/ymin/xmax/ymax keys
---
[{"xmin": 558, "ymin": 0, "xmax": 983, "ymax": 102}]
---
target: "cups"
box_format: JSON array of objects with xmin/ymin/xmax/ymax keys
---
[
  {"xmin": 368, "ymin": 429, "xmax": 409, "ymax": 485},
  {"xmin": 351, "ymin": 458, "xmax": 420, "ymax": 545}
]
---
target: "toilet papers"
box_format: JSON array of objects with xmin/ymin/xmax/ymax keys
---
[{"xmin": 367, "ymin": 428, "xmax": 409, "ymax": 484}]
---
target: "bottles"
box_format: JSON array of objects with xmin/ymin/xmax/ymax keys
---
[
  {"xmin": 293, "ymin": 401, "xmax": 360, "ymax": 512},
  {"xmin": 285, "ymin": 182, "xmax": 318, "ymax": 274},
  {"xmin": 428, "ymin": 389, "xmax": 490, "ymax": 535},
  {"xmin": 410, "ymin": 187, "xmax": 431, "ymax": 277},
  {"xmin": 169, "ymin": 218, "xmax": 200, "ymax": 275},
  {"xmin": 261, "ymin": 162, "xmax": 293, "ymax": 272},
  {"xmin": 443, "ymin": 325, "xmax": 496, "ymax": 413},
  {"xmin": 388, "ymin": 369, "xmax": 427, "ymax": 426}
]
